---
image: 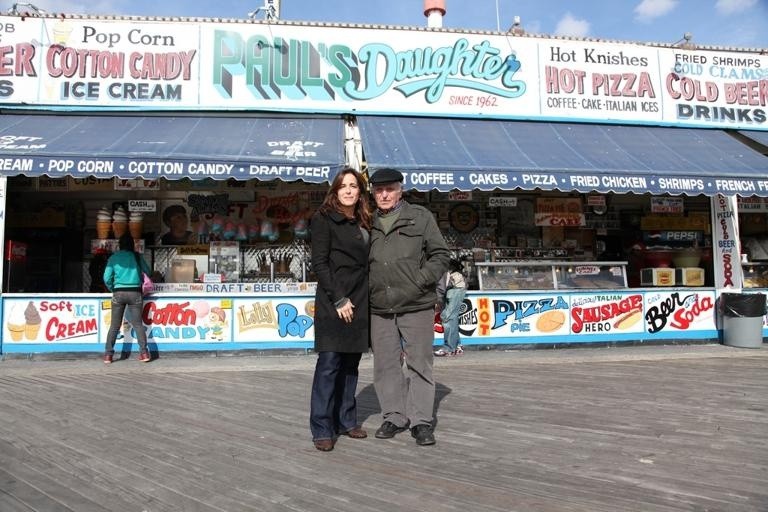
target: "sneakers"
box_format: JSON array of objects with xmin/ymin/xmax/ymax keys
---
[
  {"xmin": 104, "ymin": 355, "xmax": 112, "ymax": 364},
  {"xmin": 434, "ymin": 345, "xmax": 464, "ymax": 356},
  {"xmin": 138, "ymin": 352, "xmax": 150, "ymax": 362}
]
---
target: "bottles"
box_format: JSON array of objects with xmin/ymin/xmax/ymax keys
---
[
  {"xmin": 232, "ymin": 271, "xmax": 239, "ymax": 284},
  {"xmin": 279, "ymin": 260, "xmax": 286, "ymax": 275}
]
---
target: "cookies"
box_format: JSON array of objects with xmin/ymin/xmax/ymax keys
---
[{"xmin": 535, "ymin": 311, "xmax": 565, "ymax": 332}]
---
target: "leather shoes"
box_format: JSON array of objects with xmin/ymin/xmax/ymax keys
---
[
  {"xmin": 412, "ymin": 424, "xmax": 435, "ymax": 445},
  {"xmin": 314, "ymin": 440, "xmax": 333, "ymax": 451},
  {"xmin": 339, "ymin": 429, "xmax": 367, "ymax": 438},
  {"xmin": 375, "ymin": 421, "xmax": 404, "ymax": 438}
]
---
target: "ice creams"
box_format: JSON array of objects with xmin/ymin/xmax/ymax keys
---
[
  {"xmin": 112, "ymin": 205, "xmax": 127, "ymax": 239},
  {"xmin": 129, "ymin": 212, "xmax": 142, "ymax": 239},
  {"xmin": 24, "ymin": 301, "xmax": 41, "ymax": 339},
  {"xmin": 96, "ymin": 203, "xmax": 110, "ymax": 240},
  {"xmin": 7, "ymin": 300, "xmax": 27, "ymax": 340}
]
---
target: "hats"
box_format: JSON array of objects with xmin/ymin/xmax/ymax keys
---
[{"xmin": 369, "ymin": 168, "xmax": 403, "ymax": 185}]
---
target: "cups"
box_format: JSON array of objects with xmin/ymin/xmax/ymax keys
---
[{"xmin": 512, "ymin": 248, "xmax": 566, "ymax": 258}]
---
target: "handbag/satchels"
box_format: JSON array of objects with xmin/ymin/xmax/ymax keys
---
[{"xmin": 141, "ymin": 272, "xmax": 154, "ymax": 296}]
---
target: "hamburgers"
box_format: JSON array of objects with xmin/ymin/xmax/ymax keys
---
[{"xmin": 613, "ymin": 309, "xmax": 642, "ymax": 330}]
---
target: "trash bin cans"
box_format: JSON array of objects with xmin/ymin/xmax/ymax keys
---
[{"xmin": 720, "ymin": 293, "xmax": 767, "ymax": 348}]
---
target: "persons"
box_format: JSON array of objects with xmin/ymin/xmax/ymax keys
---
[
  {"xmin": 311, "ymin": 168, "xmax": 369, "ymax": 451},
  {"xmin": 102, "ymin": 234, "xmax": 152, "ymax": 363},
  {"xmin": 433, "ymin": 270, "xmax": 467, "ymax": 355},
  {"xmin": 162, "ymin": 205, "xmax": 197, "ymax": 245},
  {"xmin": 369, "ymin": 168, "xmax": 449, "ymax": 445}
]
